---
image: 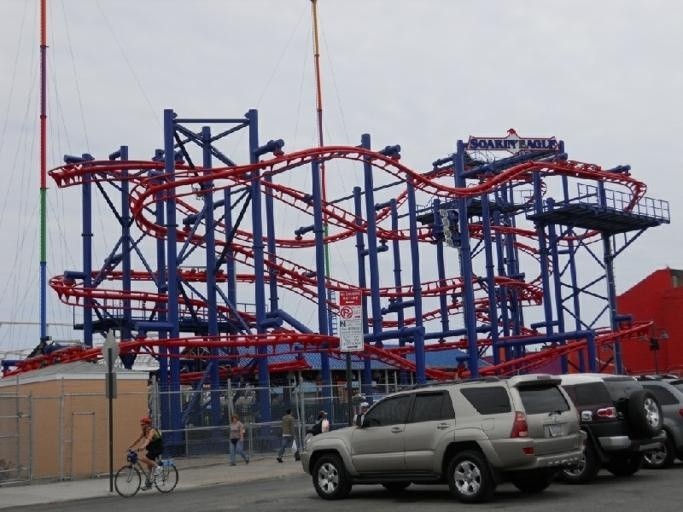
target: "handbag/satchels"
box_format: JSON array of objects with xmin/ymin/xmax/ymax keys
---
[{"xmin": 311, "ymin": 421, "xmax": 323, "ymax": 435}]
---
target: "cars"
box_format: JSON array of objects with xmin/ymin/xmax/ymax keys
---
[{"xmin": 634, "ymin": 372, "xmax": 683, "ymax": 469}]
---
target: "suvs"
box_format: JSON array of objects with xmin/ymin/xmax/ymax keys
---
[
  {"xmin": 553, "ymin": 372, "xmax": 664, "ymax": 484},
  {"xmin": 298, "ymin": 373, "xmax": 588, "ymax": 501}
]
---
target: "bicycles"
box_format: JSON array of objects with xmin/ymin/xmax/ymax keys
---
[{"xmin": 114, "ymin": 448, "xmax": 179, "ymax": 498}]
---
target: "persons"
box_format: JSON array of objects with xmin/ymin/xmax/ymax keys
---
[
  {"xmin": 353, "ymin": 402, "xmax": 370, "ymax": 426},
  {"xmin": 276, "ymin": 408, "xmax": 300, "ymax": 463},
  {"xmin": 315, "ymin": 410, "xmax": 330, "ymax": 433},
  {"xmin": 229, "ymin": 413, "xmax": 250, "ymax": 466},
  {"xmin": 128, "ymin": 417, "xmax": 164, "ymax": 490}
]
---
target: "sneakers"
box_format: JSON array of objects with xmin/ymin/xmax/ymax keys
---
[
  {"xmin": 231, "ymin": 463, "xmax": 236, "ymax": 465},
  {"xmin": 277, "ymin": 457, "xmax": 283, "ymax": 462},
  {"xmin": 154, "ymin": 466, "xmax": 164, "ymax": 476},
  {"xmin": 140, "ymin": 486, "xmax": 151, "ymax": 489},
  {"xmin": 245, "ymin": 455, "xmax": 249, "ymax": 464}
]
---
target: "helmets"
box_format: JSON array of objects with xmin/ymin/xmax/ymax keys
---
[{"xmin": 141, "ymin": 417, "xmax": 152, "ymax": 425}]
